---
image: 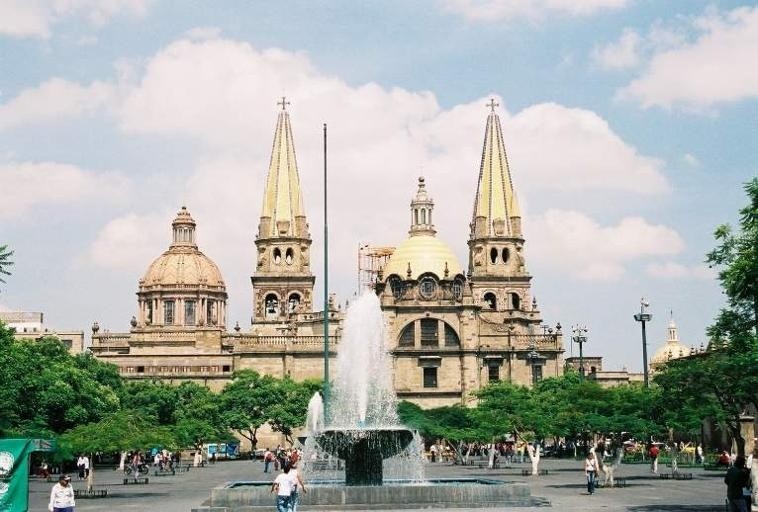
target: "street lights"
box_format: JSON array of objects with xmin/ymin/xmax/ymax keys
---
[
  {"xmin": 633, "ymin": 297, "xmax": 654, "ymax": 386},
  {"xmin": 571, "ymin": 323, "xmax": 590, "ymax": 378}
]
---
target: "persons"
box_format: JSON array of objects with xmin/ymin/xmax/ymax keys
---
[
  {"xmin": 649, "ymin": 444, "xmax": 659, "ymax": 472},
  {"xmin": 261, "ymin": 446, "xmax": 307, "ymax": 511},
  {"xmin": 428, "ymin": 440, "xmax": 534, "ymax": 465},
  {"xmin": 77, "ymin": 454, "xmax": 89, "ymax": 479},
  {"xmin": 696, "ymin": 442, "xmax": 703, "ymax": 463},
  {"xmin": 719, "ymin": 450, "xmax": 730, "ymax": 467},
  {"xmin": 585, "ymin": 442, "xmax": 614, "ymax": 496},
  {"xmin": 125, "ymin": 446, "xmax": 183, "ymax": 480},
  {"xmin": 724, "ymin": 456, "xmax": 755, "ymax": 512},
  {"xmin": 48, "ymin": 476, "xmax": 75, "ymax": 511}
]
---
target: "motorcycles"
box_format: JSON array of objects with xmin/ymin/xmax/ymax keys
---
[{"xmin": 124, "ymin": 459, "xmax": 150, "ymax": 474}]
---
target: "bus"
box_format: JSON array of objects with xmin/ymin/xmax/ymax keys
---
[{"xmin": 197, "ymin": 443, "xmax": 241, "ymax": 461}]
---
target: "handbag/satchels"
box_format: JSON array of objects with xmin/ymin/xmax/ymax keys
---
[{"xmin": 594, "ymin": 470, "xmax": 600, "ymax": 477}]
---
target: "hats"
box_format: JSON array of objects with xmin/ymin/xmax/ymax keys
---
[
  {"xmin": 281, "ymin": 448, "xmax": 285, "ymax": 451},
  {"xmin": 59, "ymin": 475, "xmax": 71, "ymax": 481}
]
